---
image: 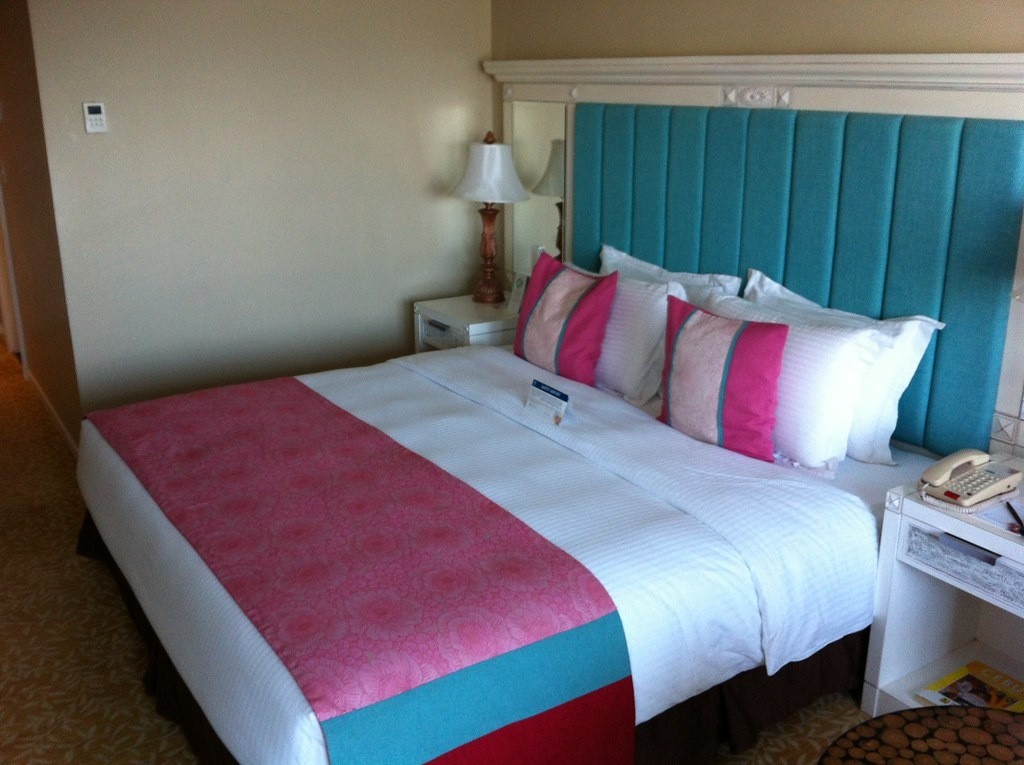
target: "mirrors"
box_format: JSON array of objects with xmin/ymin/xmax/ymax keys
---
[{"xmin": 511, "ymin": 100, "xmax": 567, "ymax": 276}]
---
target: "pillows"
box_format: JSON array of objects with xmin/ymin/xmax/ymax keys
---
[
  {"xmin": 743, "ymin": 268, "xmax": 947, "ymax": 467},
  {"xmin": 513, "ymin": 249, "xmax": 618, "ymax": 388},
  {"xmin": 707, "ymin": 289, "xmax": 898, "ymax": 481},
  {"xmin": 563, "ymin": 261, "xmax": 690, "ymax": 405},
  {"xmin": 598, "ymin": 243, "xmax": 743, "ymax": 309},
  {"xmin": 656, "ymin": 295, "xmax": 789, "ymax": 463}
]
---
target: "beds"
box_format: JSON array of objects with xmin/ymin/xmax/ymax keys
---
[{"xmin": 79, "ymin": 52, "xmax": 1023, "ymax": 765}]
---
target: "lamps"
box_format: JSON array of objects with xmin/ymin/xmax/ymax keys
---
[
  {"xmin": 449, "ymin": 131, "xmax": 531, "ymax": 304},
  {"xmin": 531, "ymin": 139, "xmax": 563, "ymax": 261}
]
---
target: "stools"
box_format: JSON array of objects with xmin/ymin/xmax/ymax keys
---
[{"xmin": 818, "ymin": 706, "xmax": 1023, "ymax": 765}]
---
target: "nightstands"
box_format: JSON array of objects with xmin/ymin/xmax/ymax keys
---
[
  {"xmin": 414, "ymin": 294, "xmax": 523, "ymax": 353},
  {"xmin": 861, "ymin": 452, "xmax": 1023, "ymax": 718}
]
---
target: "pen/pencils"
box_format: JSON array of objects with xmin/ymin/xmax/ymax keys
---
[{"xmin": 1005, "ymin": 500, "xmax": 1024, "ymax": 537}]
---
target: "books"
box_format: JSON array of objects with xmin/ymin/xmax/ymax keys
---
[{"xmin": 911, "ymin": 660, "xmax": 1024, "ymax": 715}]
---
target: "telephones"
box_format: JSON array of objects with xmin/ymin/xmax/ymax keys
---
[{"xmin": 917, "ymin": 448, "xmax": 1023, "ymax": 508}]
